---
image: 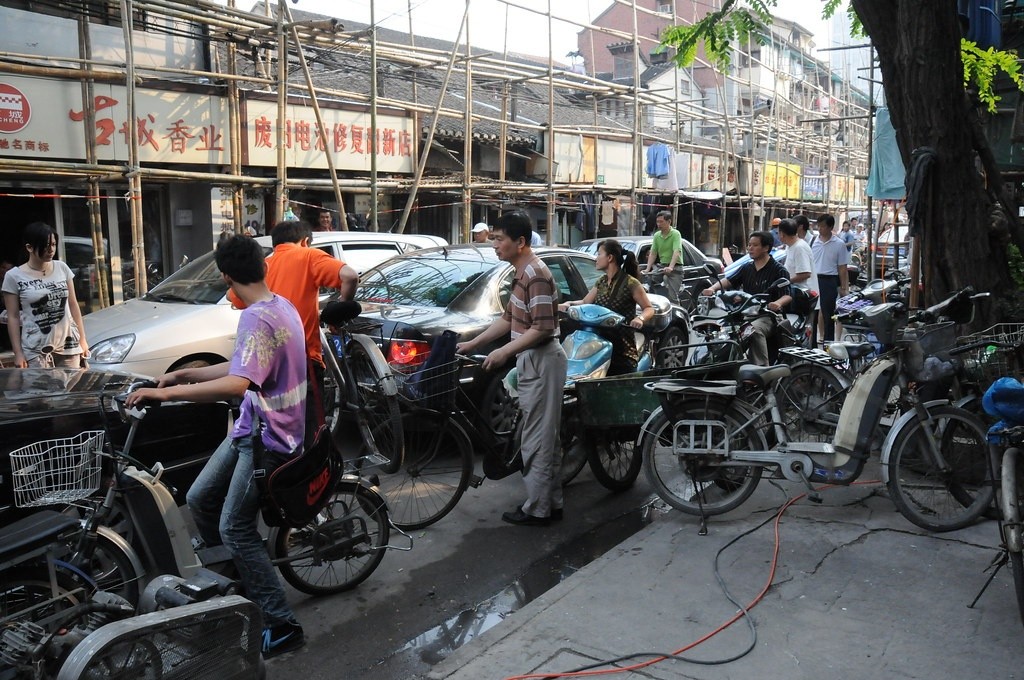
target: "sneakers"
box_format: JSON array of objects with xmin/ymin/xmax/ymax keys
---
[{"xmin": 261, "ymin": 627, "xmax": 305, "ymax": 663}]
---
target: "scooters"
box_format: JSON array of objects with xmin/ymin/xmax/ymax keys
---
[
  {"xmin": 636, "ymin": 250, "xmax": 1024, "ymax": 538},
  {"xmin": 1, "ymin": 381, "xmax": 413, "ymax": 625},
  {"xmin": 0, "ymin": 508, "xmax": 104, "ymax": 680}
]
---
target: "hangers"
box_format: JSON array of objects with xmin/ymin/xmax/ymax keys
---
[{"xmin": 653, "ymin": 138, "xmax": 661, "ymax": 145}]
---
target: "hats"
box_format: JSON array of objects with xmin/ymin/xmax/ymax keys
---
[{"xmin": 470, "ymin": 223, "xmax": 489, "ymax": 232}]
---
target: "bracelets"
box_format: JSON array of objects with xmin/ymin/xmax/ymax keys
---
[{"xmin": 841, "ymin": 286, "xmax": 850, "ymax": 290}]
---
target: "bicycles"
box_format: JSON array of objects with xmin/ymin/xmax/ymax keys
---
[
  {"xmin": 320, "ymin": 298, "xmax": 405, "ymax": 476},
  {"xmin": 948, "ymin": 322, "xmax": 1024, "ymax": 632},
  {"xmin": 119, "ymin": 254, "xmax": 164, "ymax": 301},
  {"xmin": 352, "ymin": 332, "xmax": 588, "ymax": 534}
]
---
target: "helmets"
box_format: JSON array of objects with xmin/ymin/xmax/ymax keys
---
[{"xmin": 772, "ymin": 218, "xmax": 781, "ymax": 225}]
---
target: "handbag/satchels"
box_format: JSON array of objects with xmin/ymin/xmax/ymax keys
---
[
  {"xmin": 254, "ymin": 425, "xmax": 345, "ymax": 528},
  {"xmin": 404, "ymin": 329, "xmax": 461, "ymax": 405}
]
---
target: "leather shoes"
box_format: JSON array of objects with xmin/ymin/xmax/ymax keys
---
[{"xmin": 502, "ymin": 505, "xmax": 563, "ymax": 527}]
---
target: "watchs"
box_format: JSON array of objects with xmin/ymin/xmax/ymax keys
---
[{"xmin": 637, "ymin": 315, "xmax": 645, "ymax": 323}]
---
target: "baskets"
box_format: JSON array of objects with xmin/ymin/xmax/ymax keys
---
[
  {"xmin": 895, "ymin": 321, "xmax": 961, "ymax": 382},
  {"xmin": 836, "ymin": 292, "xmax": 873, "ymax": 332},
  {"xmin": 388, "ymin": 351, "xmax": 459, "ymax": 409},
  {"xmin": 10, "ymin": 430, "xmax": 105, "ymax": 507},
  {"xmin": 956, "ymin": 323, "xmax": 1024, "ymax": 383}
]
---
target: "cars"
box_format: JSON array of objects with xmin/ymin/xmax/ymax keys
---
[
  {"xmin": 573, "ymin": 236, "xmax": 725, "ymax": 318},
  {"xmin": 0, "ymin": 367, "xmax": 241, "ymax": 595},
  {"xmin": 55, "ymin": 235, "xmax": 161, "ymax": 299},
  {"xmin": 72, "ymin": 230, "xmax": 449, "ymax": 439},
  {"xmin": 312, "ymin": 243, "xmax": 690, "ymax": 456},
  {"xmin": 869, "ymin": 226, "xmax": 911, "ymax": 279}
]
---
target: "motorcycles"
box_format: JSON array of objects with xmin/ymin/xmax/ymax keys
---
[{"xmin": 554, "ymin": 291, "xmax": 671, "ymax": 405}]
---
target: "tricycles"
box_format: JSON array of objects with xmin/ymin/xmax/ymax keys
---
[{"xmin": 574, "ymin": 290, "xmax": 775, "ymax": 498}]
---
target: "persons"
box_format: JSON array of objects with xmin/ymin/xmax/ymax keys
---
[
  {"xmin": 225, "ymin": 221, "xmax": 359, "ymax": 536},
  {"xmin": 469, "ymin": 222, "xmax": 542, "ymax": 247},
  {"xmin": 558, "ymin": 239, "xmax": 655, "ymax": 452},
  {"xmin": 0, "ymin": 259, "xmax": 16, "ymax": 282},
  {"xmin": 1, "ymin": 222, "xmax": 88, "ymax": 368},
  {"xmin": 313, "ymin": 208, "xmax": 336, "ymax": 231},
  {"xmin": 125, "ymin": 234, "xmax": 307, "ymax": 661},
  {"xmin": 769, "ymin": 213, "xmax": 892, "ymax": 351},
  {"xmin": 4, "ymin": 368, "xmax": 85, "ymax": 413},
  {"xmin": 702, "ymin": 231, "xmax": 792, "ymax": 367},
  {"xmin": 456, "ymin": 209, "xmax": 569, "ymax": 528},
  {"xmin": 640, "ymin": 211, "xmax": 684, "ymax": 306},
  {"xmin": 366, "ymin": 218, "xmax": 380, "ymax": 232}
]
